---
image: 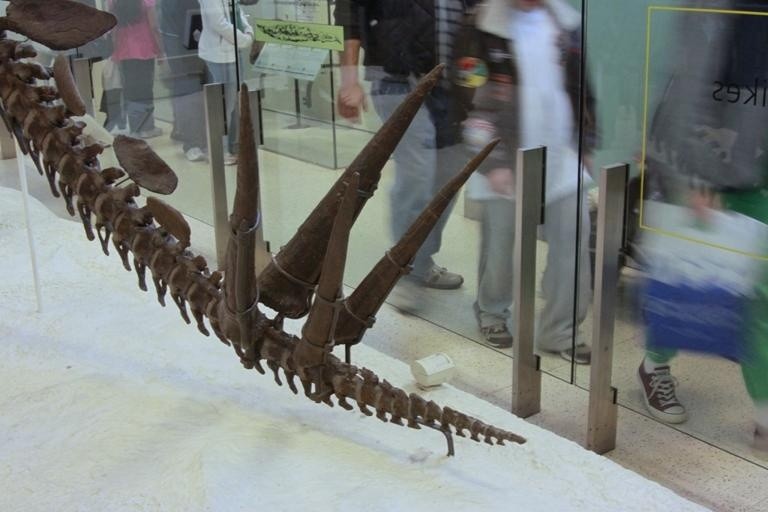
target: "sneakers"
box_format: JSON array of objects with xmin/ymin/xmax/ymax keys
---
[
  {"xmin": 638, "ymin": 356, "xmax": 687, "ymax": 424},
  {"xmin": 404, "ymin": 263, "xmax": 464, "ymax": 290},
  {"xmin": 186, "ymin": 147, "xmax": 205, "ymax": 162},
  {"xmin": 224, "ymin": 153, "xmax": 237, "ymax": 166},
  {"xmin": 560, "ymin": 342, "xmax": 592, "ymax": 364},
  {"xmin": 142, "ymin": 127, "xmax": 163, "ymax": 138},
  {"xmin": 472, "ymin": 300, "xmax": 513, "ymax": 348}
]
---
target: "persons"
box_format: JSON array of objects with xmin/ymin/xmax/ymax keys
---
[
  {"xmin": 637, "ymin": 0, "xmax": 768, "ymax": 454},
  {"xmin": 334, "ymin": 0, "xmax": 465, "ymax": 317},
  {"xmin": 156, "ymin": 0, "xmax": 210, "ymax": 163},
  {"xmin": 107, "ymin": 0, "xmax": 164, "ymax": 139},
  {"xmin": 447, "ymin": 0, "xmax": 604, "ymax": 367},
  {"xmin": 196, "ymin": 0, "xmax": 255, "ymax": 167}
]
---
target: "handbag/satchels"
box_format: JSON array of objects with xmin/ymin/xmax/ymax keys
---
[{"xmin": 640, "ymin": 199, "xmax": 767, "ymax": 365}]
